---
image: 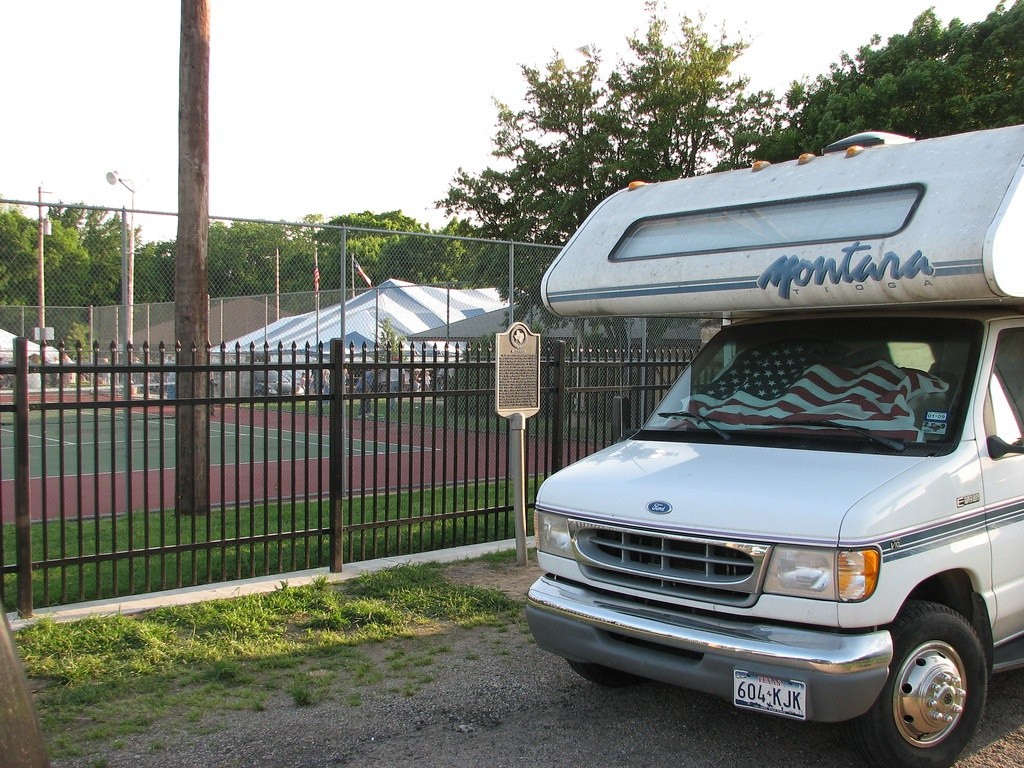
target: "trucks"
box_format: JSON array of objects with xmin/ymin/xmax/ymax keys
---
[{"xmin": 525, "ymin": 125, "xmax": 1024, "ymax": 768}]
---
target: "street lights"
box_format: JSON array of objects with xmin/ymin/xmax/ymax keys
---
[{"xmin": 107, "ymin": 171, "xmax": 135, "ymax": 385}]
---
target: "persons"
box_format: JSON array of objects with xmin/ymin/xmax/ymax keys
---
[
  {"xmin": 356, "ymin": 368, "xmax": 379, "ymax": 419},
  {"xmin": 102, "ymin": 356, "xmax": 177, "ymax": 386},
  {"xmin": 300, "ymin": 369, "xmax": 329, "ymax": 405},
  {"xmin": 0, "ymin": 353, "xmax": 74, "ymax": 389},
  {"xmin": 386, "ymin": 357, "xmax": 405, "ymax": 410}
]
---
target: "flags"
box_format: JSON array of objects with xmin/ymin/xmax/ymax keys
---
[{"xmin": 680, "ymin": 337, "xmax": 947, "ymax": 441}]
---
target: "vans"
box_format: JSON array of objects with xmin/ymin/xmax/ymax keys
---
[{"xmin": 214, "ymin": 363, "xmax": 303, "ymax": 403}]
